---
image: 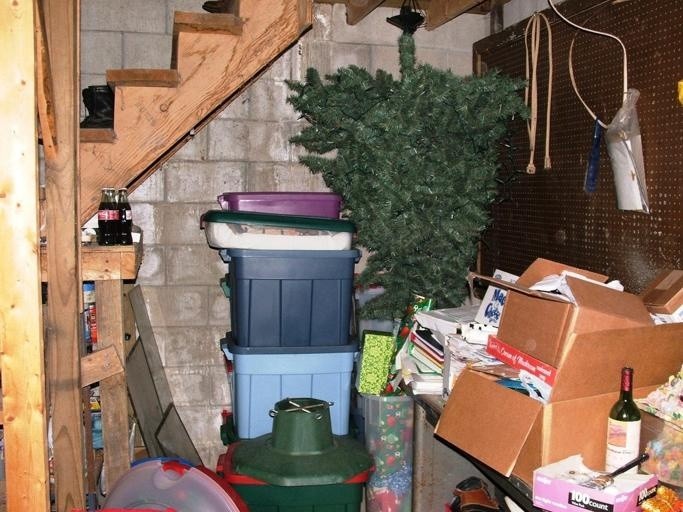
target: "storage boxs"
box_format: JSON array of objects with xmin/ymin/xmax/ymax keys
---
[
  {"xmin": 476, "ymin": 259, "xmax": 656, "ymax": 383},
  {"xmin": 216, "ymin": 397, "xmax": 373, "ymax": 512},
  {"xmin": 220, "ymin": 248, "xmax": 361, "ymax": 350},
  {"xmin": 434, "ymin": 322, "xmax": 683, "ymax": 487},
  {"xmin": 199, "ymin": 210, "xmax": 355, "ymax": 253},
  {"xmin": 218, "ymin": 191, "xmax": 342, "ymax": 220},
  {"xmin": 358, "ymin": 390, "xmax": 412, "ymax": 511},
  {"xmin": 220, "ymin": 334, "xmax": 357, "ymax": 440}
]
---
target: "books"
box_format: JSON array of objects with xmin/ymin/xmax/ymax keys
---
[{"xmin": 406, "ymin": 328, "xmax": 445, "ymax": 394}]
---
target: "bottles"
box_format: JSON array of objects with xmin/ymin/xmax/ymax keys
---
[
  {"xmin": 97, "ymin": 186, "xmax": 133, "ymax": 245},
  {"xmin": 603, "ymin": 366, "xmax": 640, "ymax": 479}
]
